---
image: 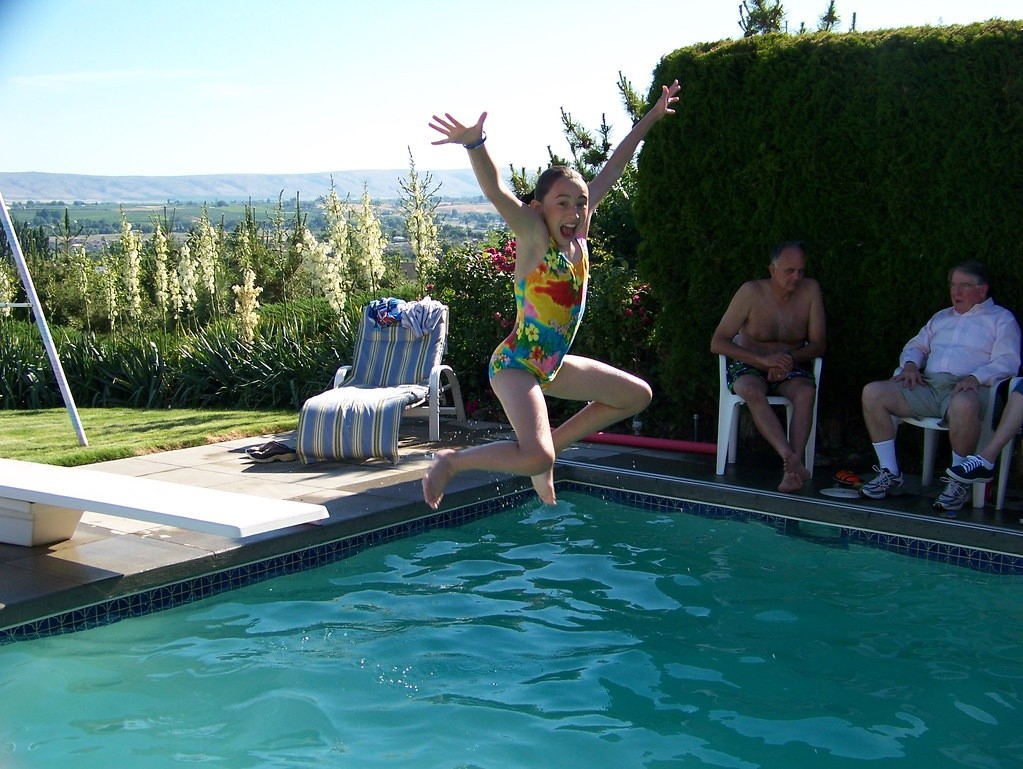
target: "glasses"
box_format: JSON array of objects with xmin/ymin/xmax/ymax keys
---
[{"xmin": 947, "ymin": 282, "xmax": 983, "ymax": 290}]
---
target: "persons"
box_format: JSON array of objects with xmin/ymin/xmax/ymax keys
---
[
  {"xmin": 860, "ymin": 258, "xmax": 1021, "ymax": 510},
  {"xmin": 946, "ymin": 379, "xmax": 1023, "ymax": 484},
  {"xmin": 421, "ymin": 78, "xmax": 681, "ymax": 509},
  {"xmin": 711, "ymin": 243, "xmax": 823, "ymax": 490}
]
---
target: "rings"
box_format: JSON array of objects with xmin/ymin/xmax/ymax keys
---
[{"xmin": 777, "ymin": 375, "xmax": 781, "ymax": 377}]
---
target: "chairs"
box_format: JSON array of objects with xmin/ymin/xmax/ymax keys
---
[
  {"xmin": 298, "ymin": 295, "xmax": 467, "ymax": 467},
  {"xmin": 716, "ymin": 335, "xmax": 823, "ymax": 478},
  {"xmin": 997, "ymin": 376, "xmax": 1023, "ymax": 510},
  {"xmin": 892, "ymin": 365, "xmax": 1010, "ymax": 508}
]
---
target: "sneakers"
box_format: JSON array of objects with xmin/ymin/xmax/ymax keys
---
[
  {"xmin": 933, "ymin": 477, "xmax": 970, "ymax": 510},
  {"xmin": 245, "ymin": 441, "xmax": 288, "ymax": 456},
  {"xmin": 946, "ymin": 455, "xmax": 994, "ymax": 483},
  {"xmin": 858, "ymin": 465, "xmax": 902, "ymax": 500},
  {"xmin": 249, "ymin": 445, "xmax": 296, "ymax": 463}
]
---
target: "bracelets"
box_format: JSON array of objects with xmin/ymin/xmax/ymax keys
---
[{"xmin": 462, "ymin": 132, "xmax": 487, "ymax": 149}]
